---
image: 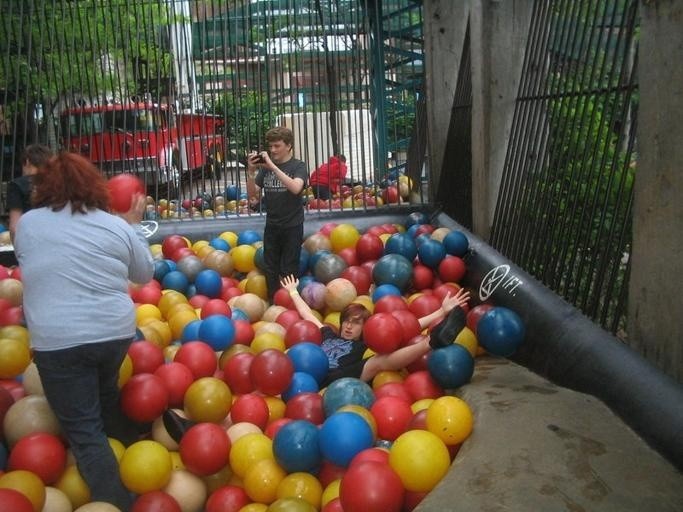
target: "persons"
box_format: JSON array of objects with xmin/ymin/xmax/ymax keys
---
[
  {"xmin": 13, "ymin": 150, "xmax": 155, "ymax": 512},
  {"xmin": 246, "ymin": 126, "xmax": 307, "ymax": 304},
  {"xmin": 160, "ymin": 273, "xmax": 473, "ymax": 450},
  {"xmin": 309, "ymin": 154, "xmax": 347, "ymax": 200},
  {"xmin": 4, "ymin": 142, "xmax": 53, "ymax": 247}
]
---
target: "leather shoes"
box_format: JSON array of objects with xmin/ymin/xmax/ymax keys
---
[
  {"xmin": 164, "ymin": 407, "xmax": 192, "ymax": 443},
  {"xmin": 429, "ymin": 306, "xmax": 467, "ymax": 349}
]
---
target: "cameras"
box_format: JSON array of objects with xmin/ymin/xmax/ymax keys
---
[{"xmin": 251, "ymin": 153, "xmax": 265, "ymax": 164}]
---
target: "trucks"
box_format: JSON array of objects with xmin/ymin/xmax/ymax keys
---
[{"xmin": 47, "ymin": 0, "xmax": 228, "ymax": 202}]
